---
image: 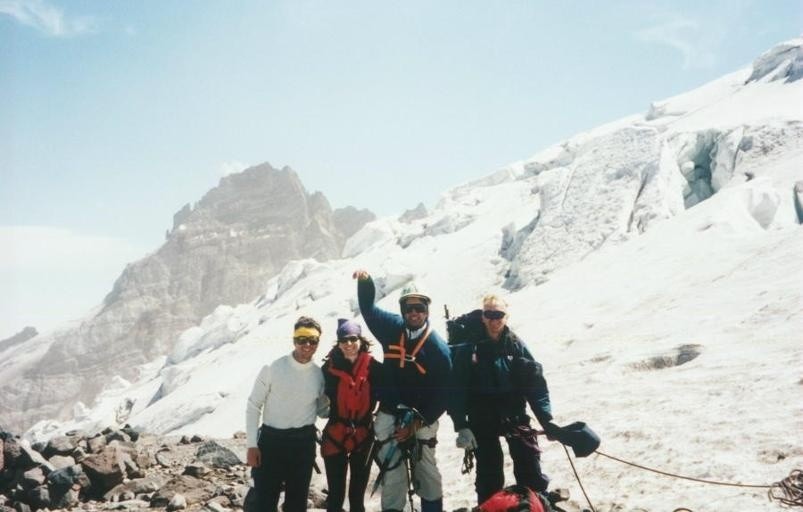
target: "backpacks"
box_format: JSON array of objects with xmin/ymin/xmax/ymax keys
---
[
  {"xmin": 447, "ymin": 310, "xmax": 483, "ymax": 342},
  {"xmin": 455, "ymin": 484, "xmax": 565, "ymax": 512}
]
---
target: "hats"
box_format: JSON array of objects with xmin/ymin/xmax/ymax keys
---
[
  {"xmin": 337, "ymin": 317, "xmax": 361, "ymax": 336},
  {"xmin": 400, "ymin": 283, "xmax": 431, "ymax": 304}
]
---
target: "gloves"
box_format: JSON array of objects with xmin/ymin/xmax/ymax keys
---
[
  {"xmin": 546, "ymin": 423, "xmax": 561, "ymax": 441},
  {"xmin": 393, "ymin": 418, "xmax": 422, "ymax": 441},
  {"xmin": 318, "ymin": 396, "xmax": 332, "ymax": 418},
  {"xmin": 456, "ymin": 428, "xmax": 478, "ymax": 450},
  {"xmin": 353, "ymin": 270, "xmax": 369, "ymax": 280}
]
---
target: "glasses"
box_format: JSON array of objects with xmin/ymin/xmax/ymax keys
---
[
  {"xmin": 403, "ymin": 304, "xmax": 427, "ymax": 312},
  {"xmin": 482, "ymin": 310, "xmax": 507, "ymax": 320},
  {"xmin": 339, "ymin": 336, "xmax": 360, "ymax": 343},
  {"xmin": 294, "ymin": 336, "xmax": 319, "ymax": 345}
]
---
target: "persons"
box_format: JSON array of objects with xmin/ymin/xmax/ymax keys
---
[
  {"xmin": 448, "ymin": 294, "xmax": 558, "ymax": 506},
  {"xmin": 242, "ymin": 316, "xmax": 329, "ymax": 512},
  {"xmin": 321, "ymin": 318, "xmax": 381, "ymax": 512},
  {"xmin": 353, "ymin": 271, "xmax": 451, "ymax": 512}
]
